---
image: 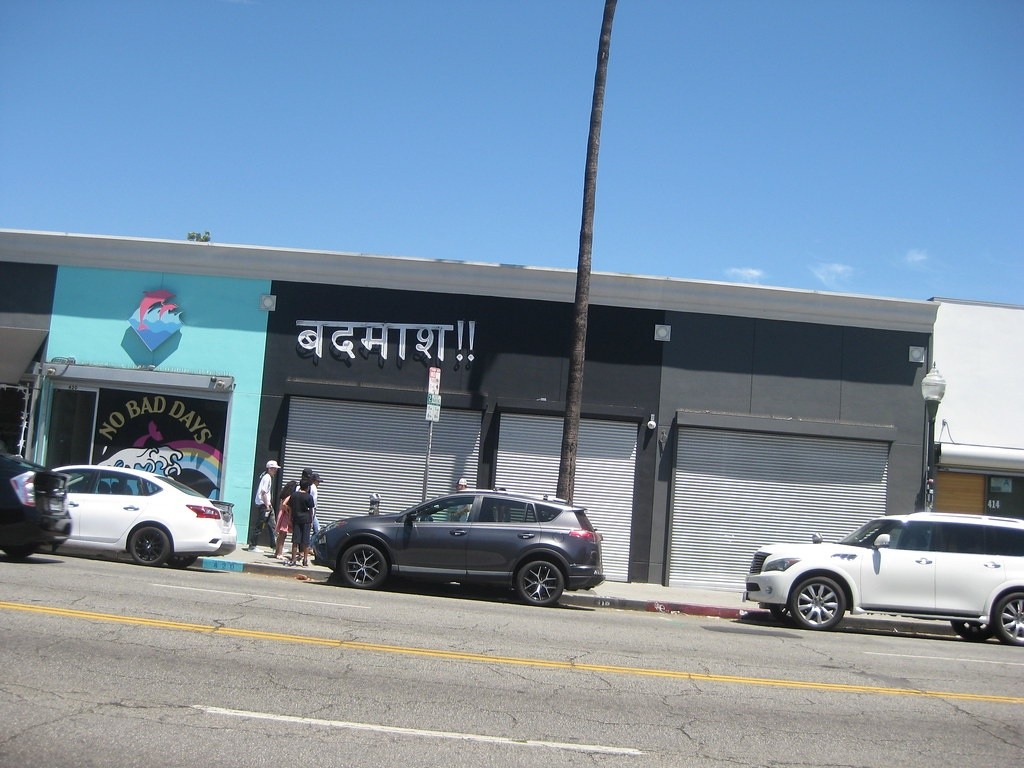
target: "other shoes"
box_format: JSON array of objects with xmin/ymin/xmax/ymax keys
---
[
  {"xmin": 248, "ymin": 547, "xmax": 265, "ymax": 553},
  {"xmin": 273, "ymin": 548, "xmax": 289, "ymax": 554},
  {"xmin": 288, "ymin": 561, "xmax": 296, "ymax": 565},
  {"xmin": 301, "ymin": 560, "xmax": 307, "ymax": 566}
]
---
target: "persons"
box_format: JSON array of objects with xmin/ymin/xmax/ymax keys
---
[
  {"xmin": 274, "ymin": 468, "xmax": 324, "ymax": 567},
  {"xmin": 450, "ymin": 478, "xmax": 472, "ymax": 523},
  {"xmin": 248, "ymin": 460, "xmax": 289, "ymax": 553}
]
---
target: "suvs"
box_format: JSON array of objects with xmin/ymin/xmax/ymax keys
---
[
  {"xmin": 739, "ymin": 509, "xmax": 1023, "ymax": 649},
  {"xmin": 308, "ymin": 488, "xmax": 608, "ymax": 607}
]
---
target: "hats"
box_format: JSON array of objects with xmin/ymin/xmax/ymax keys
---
[
  {"xmin": 312, "ymin": 474, "xmax": 324, "ymax": 482},
  {"xmin": 266, "ymin": 460, "xmax": 281, "ymax": 468},
  {"xmin": 454, "ymin": 478, "xmax": 467, "ymax": 486}
]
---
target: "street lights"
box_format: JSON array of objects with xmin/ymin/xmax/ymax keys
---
[{"xmin": 918, "ymin": 361, "xmax": 947, "ymax": 550}]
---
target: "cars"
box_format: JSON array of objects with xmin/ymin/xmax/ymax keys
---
[
  {"xmin": 0, "ymin": 440, "xmax": 73, "ymax": 559},
  {"xmin": 34, "ymin": 460, "xmax": 238, "ymax": 569}
]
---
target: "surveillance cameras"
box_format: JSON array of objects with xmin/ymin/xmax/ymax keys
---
[{"xmin": 647, "ymin": 421, "xmax": 656, "ymax": 429}]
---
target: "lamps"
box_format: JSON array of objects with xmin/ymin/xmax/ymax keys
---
[{"xmin": 921, "ymin": 361, "xmax": 946, "ymax": 426}]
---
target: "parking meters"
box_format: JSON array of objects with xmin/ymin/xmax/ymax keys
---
[
  {"xmin": 368, "ymin": 492, "xmax": 381, "ymax": 516},
  {"xmin": 812, "ymin": 532, "xmax": 823, "ymax": 544}
]
